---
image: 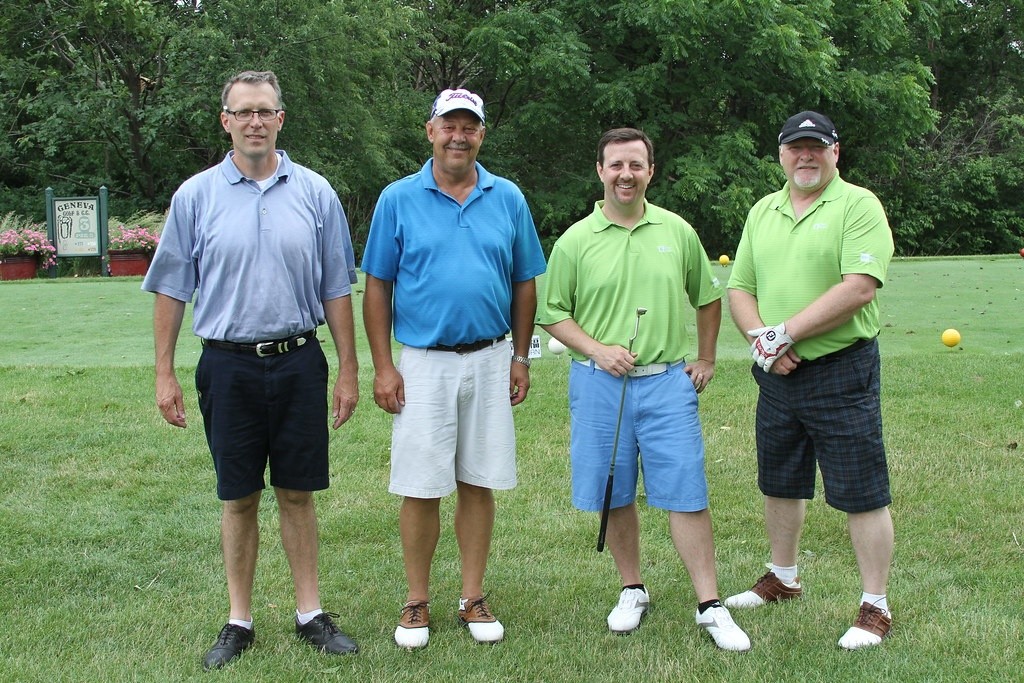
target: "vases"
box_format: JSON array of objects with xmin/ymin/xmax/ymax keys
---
[
  {"xmin": 107, "ymin": 248, "xmax": 149, "ymax": 278},
  {"xmin": 0, "ymin": 253, "xmax": 36, "ymax": 280}
]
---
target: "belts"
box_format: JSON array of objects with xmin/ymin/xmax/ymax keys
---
[
  {"xmin": 204, "ymin": 329, "xmax": 318, "ymax": 355},
  {"xmin": 429, "ymin": 335, "xmax": 505, "ymax": 353},
  {"xmin": 574, "ymin": 357, "xmax": 684, "ymax": 377}
]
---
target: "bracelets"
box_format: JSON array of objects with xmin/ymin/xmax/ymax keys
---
[{"xmin": 511, "ymin": 355, "xmax": 531, "ymax": 369}]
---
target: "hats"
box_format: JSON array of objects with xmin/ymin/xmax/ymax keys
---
[
  {"xmin": 778, "ymin": 111, "xmax": 838, "ymax": 145},
  {"xmin": 429, "ymin": 88, "xmax": 486, "ymax": 124}
]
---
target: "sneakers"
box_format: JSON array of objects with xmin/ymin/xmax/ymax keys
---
[
  {"xmin": 606, "ymin": 584, "xmax": 650, "ymax": 634},
  {"xmin": 457, "ymin": 589, "xmax": 505, "ymax": 644},
  {"xmin": 723, "ymin": 569, "xmax": 803, "ymax": 609},
  {"xmin": 295, "ymin": 611, "xmax": 359, "ymax": 655},
  {"xmin": 203, "ymin": 623, "xmax": 255, "ymax": 673},
  {"xmin": 838, "ymin": 596, "xmax": 892, "ymax": 651},
  {"xmin": 394, "ymin": 600, "xmax": 430, "ymax": 650},
  {"xmin": 695, "ymin": 602, "xmax": 751, "ymax": 651}
]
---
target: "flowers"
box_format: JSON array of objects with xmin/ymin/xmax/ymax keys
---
[
  {"xmin": 0, "ymin": 226, "xmax": 58, "ymax": 270},
  {"xmin": 101, "ymin": 223, "xmax": 160, "ymax": 276}
]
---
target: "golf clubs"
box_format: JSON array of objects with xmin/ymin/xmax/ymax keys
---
[{"xmin": 595, "ymin": 306, "xmax": 649, "ymax": 551}]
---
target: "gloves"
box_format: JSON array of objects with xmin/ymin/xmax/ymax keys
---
[{"xmin": 747, "ymin": 322, "xmax": 796, "ymax": 373}]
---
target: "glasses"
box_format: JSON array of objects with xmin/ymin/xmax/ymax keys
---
[{"xmin": 227, "ymin": 108, "xmax": 281, "ymax": 122}]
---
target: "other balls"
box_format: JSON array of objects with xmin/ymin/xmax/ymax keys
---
[
  {"xmin": 719, "ymin": 254, "xmax": 729, "ymax": 265},
  {"xmin": 547, "ymin": 336, "xmax": 567, "ymax": 355},
  {"xmin": 942, "ymin": 329, "xmax": 961, "ymax": 347}
]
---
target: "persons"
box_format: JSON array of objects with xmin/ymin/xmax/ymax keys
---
[
  {"xmin": 723, "ymin": 110, "xmax": 896, "ymax": 651},
  {"xmin": 532, "ymin": 128, "xmax": 751, "ymax": 652},
  {"xmin": 139, "ymin": 68, "xmax": 360, "ymax": 672},
  {"xmin": 360, "ymin": 89, "xmax": 548, "ymax": 649}
]
